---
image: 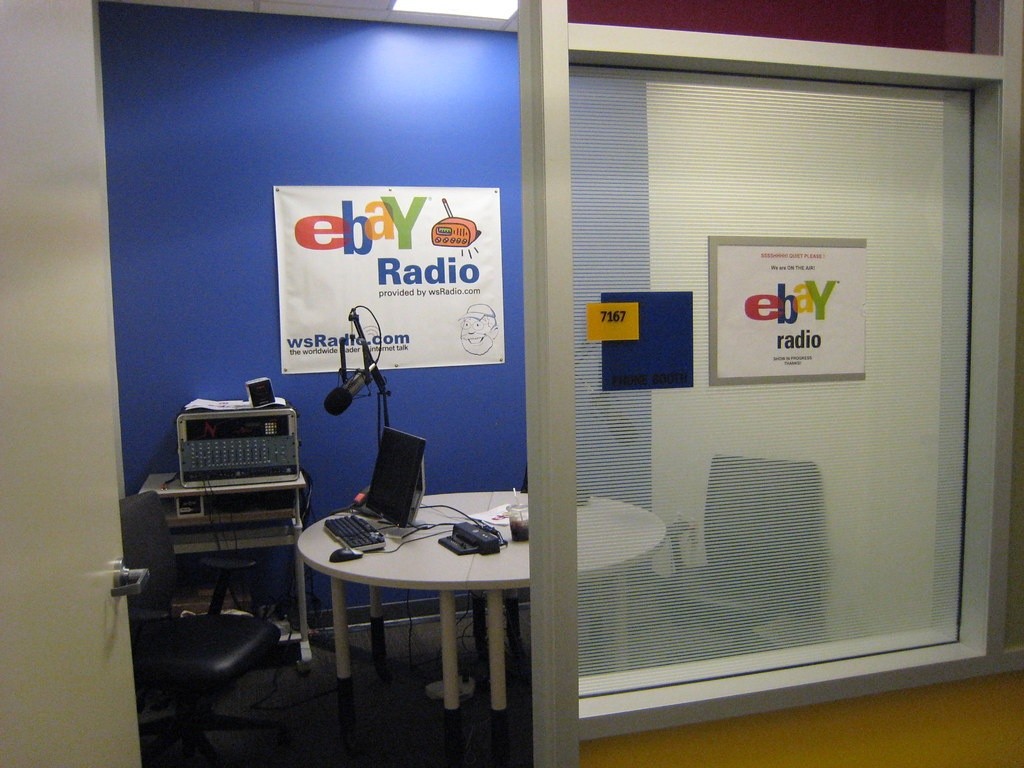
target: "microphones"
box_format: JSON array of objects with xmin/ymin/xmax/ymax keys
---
[{"xmin": 324, "ymin": 362, "xmax": 377, "ymax": 416}]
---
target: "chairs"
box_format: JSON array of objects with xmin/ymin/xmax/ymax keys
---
[
  {"xmin": 120, "ymin": 488, "xmax": 291, "ymax": 768},
  {"xmin": 655, "ymin": 454, "xmax": 821, "ymax": 654},
  {"xmin": 295, "ymin": 492, "xmax": 666, "ymax": 767}
]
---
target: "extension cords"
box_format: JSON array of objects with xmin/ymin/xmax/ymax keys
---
[{"xmin": 425, "ymin": 676, "xmax": 475, "ymax": 700}]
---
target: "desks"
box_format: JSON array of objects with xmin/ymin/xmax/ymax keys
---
[{"xmin": 138, "ymin": 471, "xmax": 313, "ymax": 675}]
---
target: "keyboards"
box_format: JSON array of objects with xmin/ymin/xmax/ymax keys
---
[{"xmin": 324, "ymin": 515, "xmax": 386, "ymax": 552}]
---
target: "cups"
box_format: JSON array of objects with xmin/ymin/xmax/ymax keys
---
[{"xmin": 506, "ymin": 504, "xmax": 529, "ymax": 542}]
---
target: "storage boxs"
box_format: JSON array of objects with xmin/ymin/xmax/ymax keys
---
[{"xmin": 170, "ymin": 583, "xmax": 247, "ymax": 618}]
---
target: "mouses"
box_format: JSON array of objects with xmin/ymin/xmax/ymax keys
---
[{"xmin": 329, "ymin": 548, "xmax": 363, "ymax": 562}]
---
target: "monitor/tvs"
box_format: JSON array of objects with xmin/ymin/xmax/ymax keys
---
[{"xmin": 366, "ymin": 425, "xmax": 429, "ymax": 539}]
